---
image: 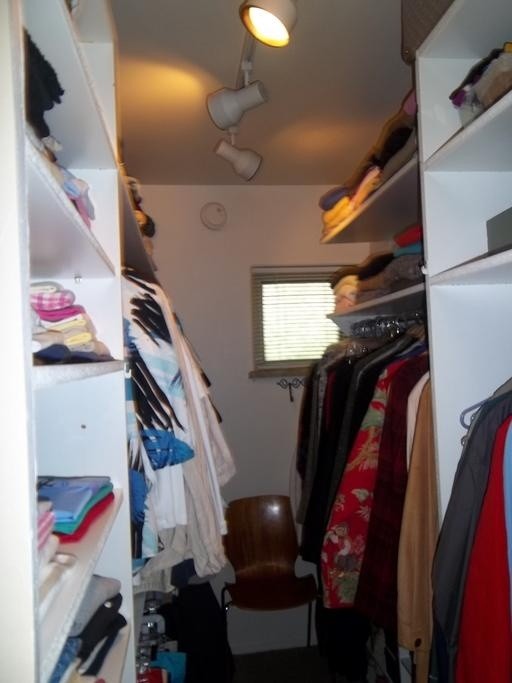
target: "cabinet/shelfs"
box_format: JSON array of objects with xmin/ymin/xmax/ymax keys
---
[
  {"xmin": 0, "ymin": 0, "xmax": 179, "ymax": 683},
  {"xmin": 320, "ymin": 0, "xmax": 512, "ymax": 683}
]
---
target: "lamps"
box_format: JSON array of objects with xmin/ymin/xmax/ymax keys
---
[{"xmin": 205, "ymin": 0, "xmax": 298, "ymax": 180}]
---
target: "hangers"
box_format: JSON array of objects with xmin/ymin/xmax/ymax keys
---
[
  {"xmin": 344, "ymin": 312, "xmax": 427, "ymax": 340},
  {"xmin": 459, "ymin": 400, "xmax": 491, "ymax": 428}
]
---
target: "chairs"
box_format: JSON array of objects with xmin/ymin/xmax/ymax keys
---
[{"xmin": 222, "ymin": 495, "xmax": 316, "ymax": 683}]
